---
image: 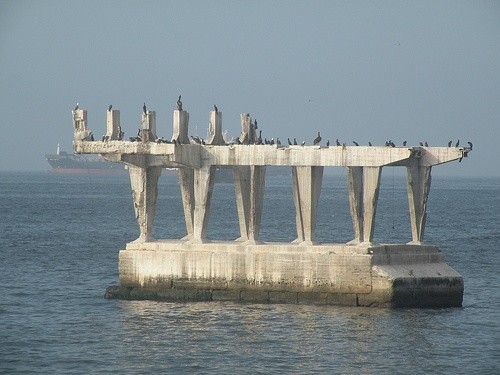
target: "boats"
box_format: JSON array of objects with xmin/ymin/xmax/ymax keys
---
[{"xmin": 44, "ymin": 143, "xmax": 131, "ymax": 176}]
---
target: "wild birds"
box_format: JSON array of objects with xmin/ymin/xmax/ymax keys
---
[
  {"xmin": 107, "ymin": 104, "xmax": 113, "ymax": 112},
  {"xmin": 142, "ymin": 102, "xmax": 148, "ymax": 116},
  {"xmin": 117, "ymin": 125, "xmax": 125, "ymax": 140},
  {"xmin": 293, "ymin": 137, "xmax": 297, "ymax": 145},
  {"xmin": 74, "ymin": 102, "xmax": 81, "ymax": 111},
  {"xmin": 336, "ymin": 138, "xmax": 341, "ymax": 147},
  {"xmin": 287, "ymin": 137, "xmax": 292, "ymax": 145},
  {"xmin": 255, "ymin": 130, "xmax": 282, "ymax": 145},
  {"xmin": 254, "ymin": 119, "xmax": 258, "ymax": 129},
  {"xmin": 367, "ymin": 141, "xmax": 373, "ymax": 146},
  {"xmin": 214, "ymin": 103, "xmax": 218, "ymax": 114},
  {"xmin": 154, "ymin": 136, "xmax": 172, "ymax": 145},
  {"xmin": 352, "ymin": 141, "xmax": 359, "ymax": 146},
  {"xmin": 419, "ymin": 141, "xmax": 429, "ymax": 147},
  {"xmin": 176, "ymin": 94, "xmax": 184, "ymax": 112},
  {"xmin": 301, "ymin": 140, "xmax": 306, "ymax": 146},
  {"xmin": 233, "ymin": 136, "xmax": 242, "ymax": 145},
  {"xmin": 467, "ymin": 141, "xmax": 473, "ymax": 148},
  {"xmin": 385, "ymin": 139, "xmax": 395, "ymax": 148},
  {"xmin": 402, "ymin": 140, "xmax": 408, "ymax": 146},
  {"xmin": 326, "ymin": 139, "xmax": 331, "ymax": 146},
  {"xmin": 455, "ymin": 139, "xmax": 460, "ymax": 147},
  {"xmin": 447, "ymin": 140, "xmax": 453, "ymax": 147},
  {"xmin": 190, "ymin": 135, "xmax": 206, "ymax": 145},
  {"xmin": 313, "ymin": 131, "xmax": 323, "ymax": 146}
]
